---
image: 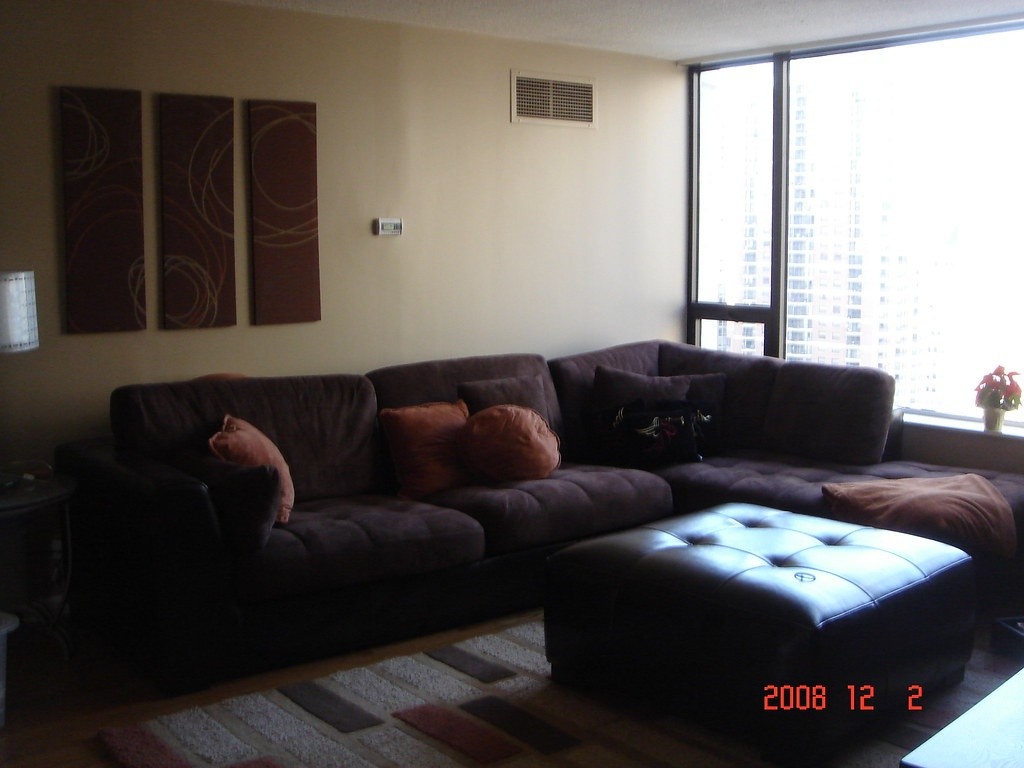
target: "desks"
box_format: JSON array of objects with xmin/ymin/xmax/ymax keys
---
[{"xmin": 898, "ymin": 669, "xmax": 1024, "ymax": 768}]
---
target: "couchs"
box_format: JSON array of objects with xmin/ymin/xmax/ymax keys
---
[
  {"xmin": 654, "ymin": 340, "xmax": 1024, "ymax": 617},
  {"xmin": 61, "ymin": 341, "xmax": 674, "ymax": 700}
]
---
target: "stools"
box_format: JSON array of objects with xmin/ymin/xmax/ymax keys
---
[{"xmin": 542, "ymin": 500, "xmax": 978, "ymax": 768}]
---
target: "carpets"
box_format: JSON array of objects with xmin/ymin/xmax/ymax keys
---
[{"xmin": 92, "ymin": 614, "xmax": 1024, "ymax": 768}]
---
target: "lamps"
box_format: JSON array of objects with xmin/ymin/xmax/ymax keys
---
[{"xmin": 0, "ymin": 269, "xmax": 39, "ymax": 355}]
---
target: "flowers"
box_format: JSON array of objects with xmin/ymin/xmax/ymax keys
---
[{"xmin": 972, "ymin": 364, "xmax": 1023, "ymax": 410}]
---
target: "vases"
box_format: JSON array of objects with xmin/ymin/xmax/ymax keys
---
[{"xmin": 983, "ymin": 407, "xmax": 1006, "ymax": 431}]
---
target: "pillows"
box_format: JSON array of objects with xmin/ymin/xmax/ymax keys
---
[
  {"xmin": 456, "ymin": 373, "xmax": 551, "ymax": 426},
  {"xmin": 590, "ymin": 364, "xmax": 727, "ymax": 458},
  {"xmin": 580, "ymin": 399, "xmax": 647, "ymax": 465},
  {"xmin": 173, "ymin": 446, "xmax": 282, "ymax": 557},
  {"xmin": 206, "ymin": 412, "xmax": 296, "ymax": 525},
  {"xmin": 622, "ymin": 411, "xmax": 699, "ymax": 467},
  {"xmin": 455, "ymin": 403, "xmax": 563, "ymax": 480},
  {"xmin": 378, "ymin": 399, "xmax": 484, "ymax": 501},
  {"xmin": 654, "ymin": 398, "xmax": 724, "ymax": 458}
]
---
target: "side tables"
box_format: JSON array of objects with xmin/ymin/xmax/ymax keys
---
[{"xmin": 0, "ymin": 467, "xmax": 80, "ymax": 672}]
